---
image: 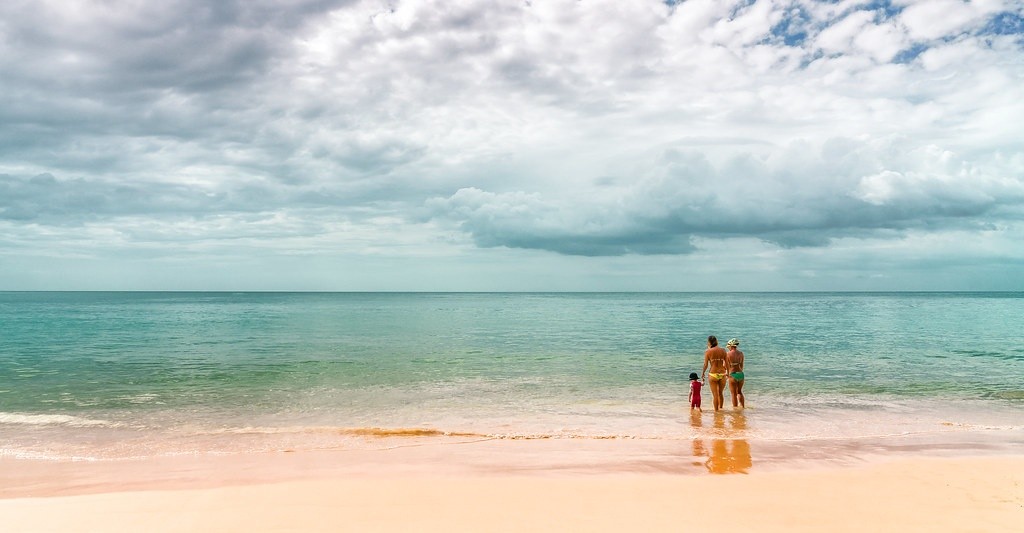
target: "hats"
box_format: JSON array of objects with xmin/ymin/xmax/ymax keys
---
[
  {"xmin": 728, "ymin": 338, "xmax": 739, "ymax": 345},
  {"xmin": 689, "ymin": 372, "xmax": 699, "ymax": 380}
]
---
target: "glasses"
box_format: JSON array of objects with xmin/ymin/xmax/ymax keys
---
[{"xmin": 726, "ymin": 345, "xmax": 728, "ymax": 348}]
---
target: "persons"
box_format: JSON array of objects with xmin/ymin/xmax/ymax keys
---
[
  {"xmin": 701, "ymin": 336, "xmax": 729, "ymax": 411},
  {"xmin": 689, "ymin": 373, "xmax": 705, "ymax": 412},
  {"xmin": 724, "ymin": 338, "xmax": 744, "ymax": 407}
]
